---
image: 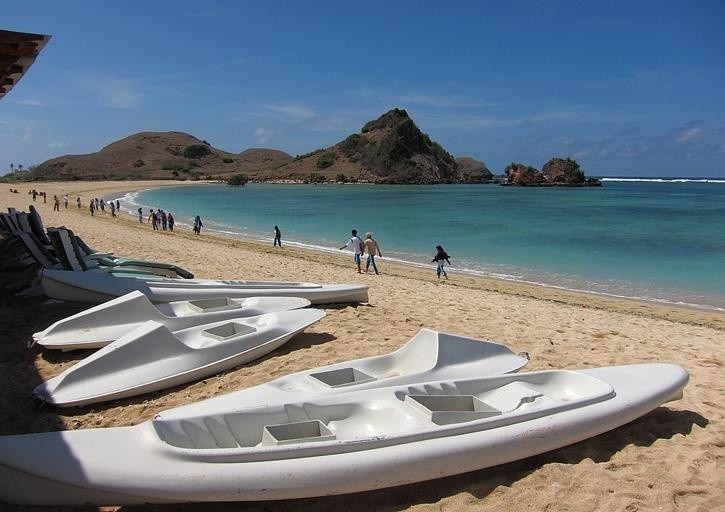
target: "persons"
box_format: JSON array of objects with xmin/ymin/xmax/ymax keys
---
[
  {"xmin": 273, "ymin": 226, "xmax": 282, "ymax": 247},
  {"xmin": 9, "ymin": 187, "xmax": 203, "ymax": 235},
  {"xmin": 340, "ymin": 228, "xmax": 364, "ymax": 273},
  {"xmin": 363, "ymin": 232, "xmax": 382, "ymax": 274},
  {"xmin": 431, "ymin": 245, "xmax": 451, "ymax": 279}
]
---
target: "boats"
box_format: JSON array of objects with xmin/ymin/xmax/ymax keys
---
[
  {"xmin": 33, "ymin": 307, "xmax": 327, "ymax": 407},
  {"xmin": 0, "ymin": 362, "xmax": 689, "ymax": 507},
  {"xmin": 32, "ymin": 290, "xmax": 312, "ymax": 352},
  {"xmin": 37, "ymin": 267, "xmax": 369, "ymax": 307},
  {"xmin": 155, "ymin": 328, "xmax": 528, "ymax": 421}
]
